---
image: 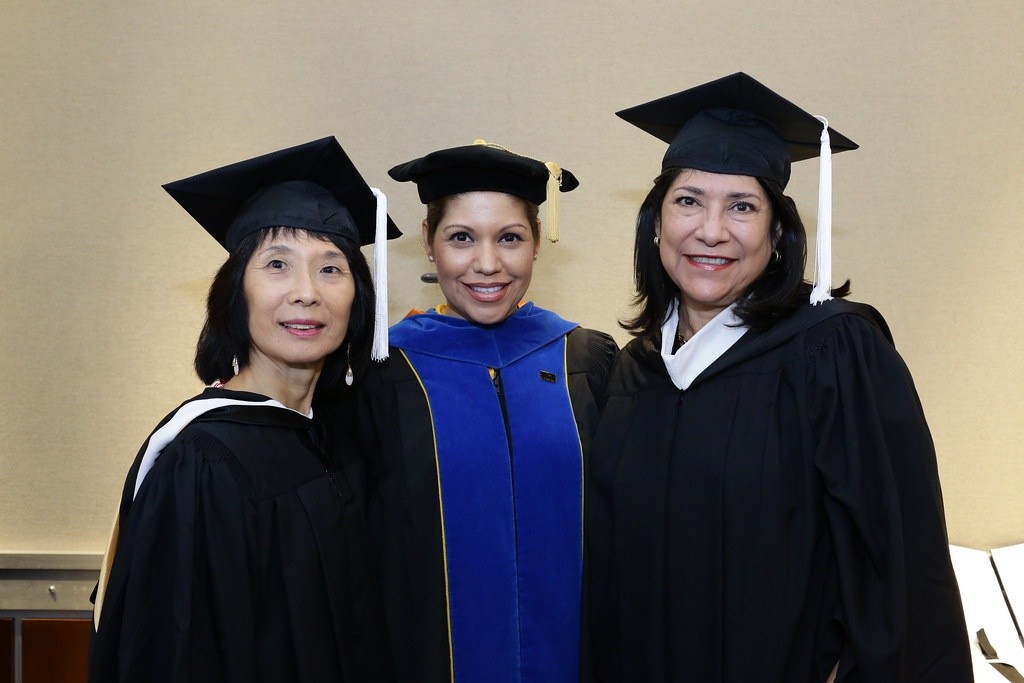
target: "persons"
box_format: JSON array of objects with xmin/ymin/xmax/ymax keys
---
[
  {"xmin": 377, "ymin": 142, "xmax": 619, "ymax": 683},
  {"xmin": 86, "ymin": 135, "xmax": 401, "ymax": 683},
  {"xmin": 585, "ymin": 72, "xmax": 974, "ymax": 683}
]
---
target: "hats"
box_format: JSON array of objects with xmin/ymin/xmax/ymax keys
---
[
  {"xmin": 386, "ymin": 137, "xmax": 581, "ymax": 244},
  {"xmin": 614, "ymin": 72, "xmax": 860, "ymax": 308},
  {"xmin": 160, "ymin": 136, "xmax": 404, "ymax": 363}
]
